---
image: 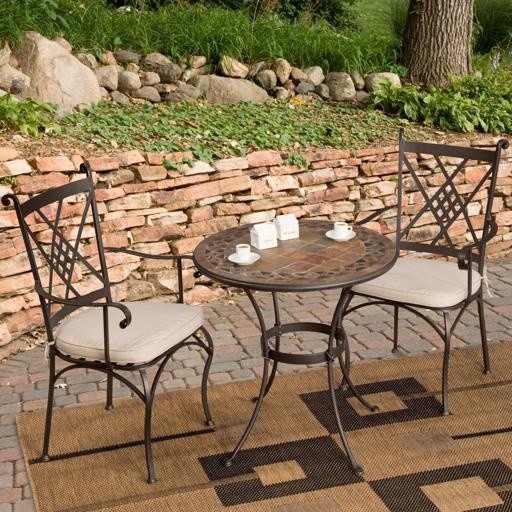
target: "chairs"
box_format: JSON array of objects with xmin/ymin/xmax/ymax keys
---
[
  {"xmin": 336, "ymin": 126, "xmax": 510, "ymax": 417},
  {"xmin": 0, "ymin": 161, "xmax": 213, "ymax": 487}
]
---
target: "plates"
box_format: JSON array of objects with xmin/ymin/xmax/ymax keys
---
[
  {"xmin": 325, "ymin": 229, "xmax": 357, "ymax": 242},
  {"xmin": 227, "ymin": 252, "xmax": 261, "ymax": 266}
]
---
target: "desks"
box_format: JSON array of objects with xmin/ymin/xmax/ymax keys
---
[{"xmin": 194, "ymin": 217, "xmax": 400, "ymax": 479}]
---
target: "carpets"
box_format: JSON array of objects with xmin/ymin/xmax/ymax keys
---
[{"xmin": 15, "ymin": 338, "xmax": 511, "ymax": 512}]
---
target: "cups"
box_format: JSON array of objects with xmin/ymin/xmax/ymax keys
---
[
  {"xmin": 235, "ymin": 243, "xmax": 251, "ymax": 259},
  {"xmin": 333, "ymin": 222, "xmax": 353, "ymax": 237}
]
